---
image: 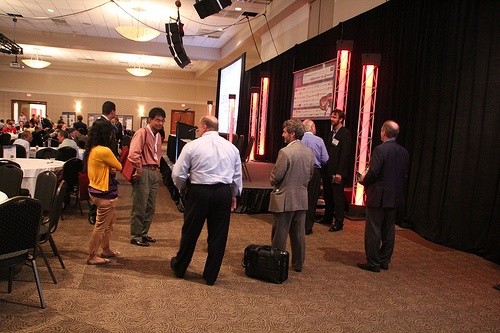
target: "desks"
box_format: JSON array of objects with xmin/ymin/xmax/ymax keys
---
[
  {"xmin": 30, "ymin": 145, "xmax": 84, "ymax": 161},
  {"xmin": 11, "ymin": 157, "xmax": 65, "ymax": 196},
  {"xmin": 0, "ymin": 191, "xmax": 9, "ymax": 204}
]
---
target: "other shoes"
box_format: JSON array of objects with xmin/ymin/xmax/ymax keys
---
[
  {"xmin": 305, "ymin": 229, "xmax": 313, "ymax": 234},
  {"xmin": 202, "ymin": 274, "xmax": 216, "ymax": 286},
  {"xmin": 357, "ymin": 262, "xmax": 380, "ymax": 272},
  {"xmin": 101, "ymin": 250, "xmax": 121, "ymax": 257},
  {"xmin": 171, "ymin": 257, "xmax": 184, "ymax": 277},
  {"xmin": 381, "ymin": 263, "xmax": 388, "ymax": 270},
  {"xmin": 87, "ymin": 257, "xmax": 111, "ymax": 265}
]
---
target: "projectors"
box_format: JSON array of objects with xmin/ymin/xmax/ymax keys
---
[{"xmin": 10, "ymin": 62, "xmax": 20, "ymax": 68}]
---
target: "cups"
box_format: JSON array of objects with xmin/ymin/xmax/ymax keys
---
[{"xmin": 50, "ymin": 158, "xmax": 55, "ymax": 163}]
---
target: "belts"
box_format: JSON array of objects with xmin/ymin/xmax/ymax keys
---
[{"xmin": 143, "ymin": 164, "xmax": 160, "ymax": 171}]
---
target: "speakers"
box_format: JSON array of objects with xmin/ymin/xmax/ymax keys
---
[
  {"xmin": 194, "ymin": 0, "xmax": 231, "ymax": 19},
  {"xmin": 165, "ymin": 23, "xmax": 191, "ymax": 68}
]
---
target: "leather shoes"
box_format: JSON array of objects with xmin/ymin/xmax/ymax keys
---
[
  {"xmin": 329, "ymin": 226, "xmax": 343, "ymax": 232},
  {"xmin": 131, "ymin": 238, "xmax": 149, "ymax": 247},
  {"xmin": 142, "ymin": 235, "xmax": 156, "ymax": 243},
  {"xmin": 318, "ymin": 219, "xmax": 333, "ymax": 224}
]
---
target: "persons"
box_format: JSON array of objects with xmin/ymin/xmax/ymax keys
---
[
  {"xmin": 89, "ymin": 100, "xmax": 117, "ymax": 224},
  {"xmin": 128, "ymin": 107, "xmax": 166, "ymax": 246},
  {"xmin": 171, "ymin": 116, "xmax": 243, "ymax": 285},
  {"xmin": 356, "ymin": 121, "xmax": 408, "ymax": 272},
  {"xmin": 87, "ymin": 121, "xmax": 123, "ymax": 265},
  {"xmin": 316, "ymin": 110, "xmax": 356, "ymax": 231},
  {"xmin": 0, "ymin": 112, "xmax": 88, "ymax": 158},
  {"xmin": 269, "ymin": 119, "xmax": 315, "ymax": 272},
  {"xmin": 301, "ymin": 119, "xmax": 329, "ymax": 235},
  {"xmin": 115, "ymin": 117, "xmax": 127, "ymax": 135}
]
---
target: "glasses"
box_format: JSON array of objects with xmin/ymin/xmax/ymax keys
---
[{"xmin": 153, "ymin": 118, "xmax": 166, "ymax": 122}]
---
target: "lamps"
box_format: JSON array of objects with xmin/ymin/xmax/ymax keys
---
[
  {"xmin": 21, "ymin": 47, "xmax": 51, "ymax": 69},
  {"xmin": 127, "ymin": 54, "xmax": 151, "ymax": 77},
  {"xmin": 246, "ymin": 75, "xmax": 271, "ymax": 162},
  {"xmin": 115, "ymin": 8, "xmax": 162, "ymax": 41},
  {"xmin": 205, "ymin": 101, "xmax": 215, "ymax": 115},
  {"xmin": 346, "ymin": 52, "xmax": 384, "ymax": 220},
  {"xmin": 164, "ymin": 0, "xmax": 190, "ymax": 69},
  {"xmin": 193, "ymin": 0, "xmax": 233, "ymax": 19},
  {"xmin": 227, "ymin": 93, "xmax": 236, "ymax": 142},
  {"xmin": 335, "ymin": 40, "xmax": 355, "ymax": 126}
]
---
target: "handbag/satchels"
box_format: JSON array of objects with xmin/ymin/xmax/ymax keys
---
[
  {"xmin": 244, "ymin": 244, "xmax": 289, "ymax": 284},
  {"xmin": 121, "ymin": 160, "xmax": 137, "ymax": 182}
]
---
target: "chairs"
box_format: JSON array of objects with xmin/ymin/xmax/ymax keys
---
[
  {"xmin": 222, "ymin": 131, "xmax": 250, "ymax": 179},
  {"xmin": 0, "ymin": 139, "xmax": 90, "ymax": 309}
]
